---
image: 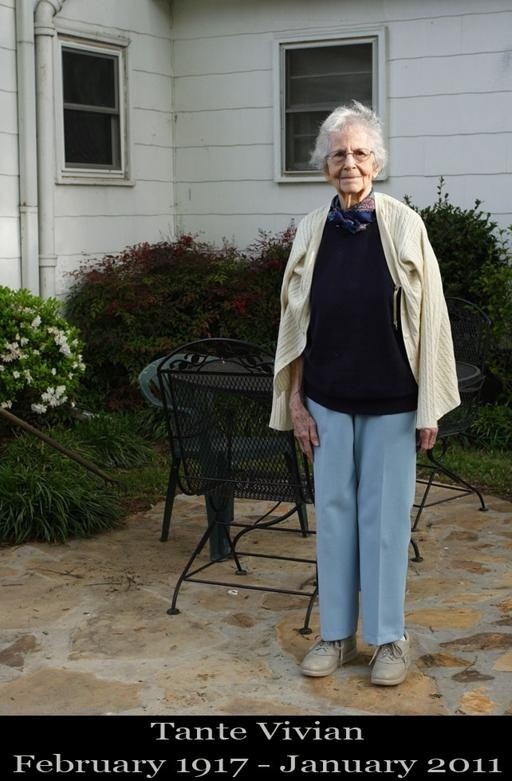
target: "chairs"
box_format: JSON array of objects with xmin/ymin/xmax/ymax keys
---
[
  {"xmin": 157, "ymin": 337, "xmax": 321, "ymax": 636},
  {"xmin": 138, "ymin": 354, "xmax": 311, "ymax": 563},
  {"xmin": 410, "ymin": 295, "xmax": 491, "ymax": 532}
]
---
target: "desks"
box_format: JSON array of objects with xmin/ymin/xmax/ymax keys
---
[{"xmin": 171, "ymin": 359, "xmax": 487, "ymax": 563}]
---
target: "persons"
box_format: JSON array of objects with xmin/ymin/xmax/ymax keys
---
[{"xmin": 267, "ymin": 101, "xmax": 464, "ymax": 686}]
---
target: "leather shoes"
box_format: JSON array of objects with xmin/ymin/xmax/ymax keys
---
[
  {"xmin": 298, "ymin": 634, "xmax": 356, "ymax": 678},
  {"xmin": 368, "ymin": 631, "xmax": 411, "ymax": 688}
]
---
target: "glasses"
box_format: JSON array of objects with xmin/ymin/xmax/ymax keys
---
[{"xmin": 327, "ymin": 147, "xmax": 373, "ymax": 163}]
